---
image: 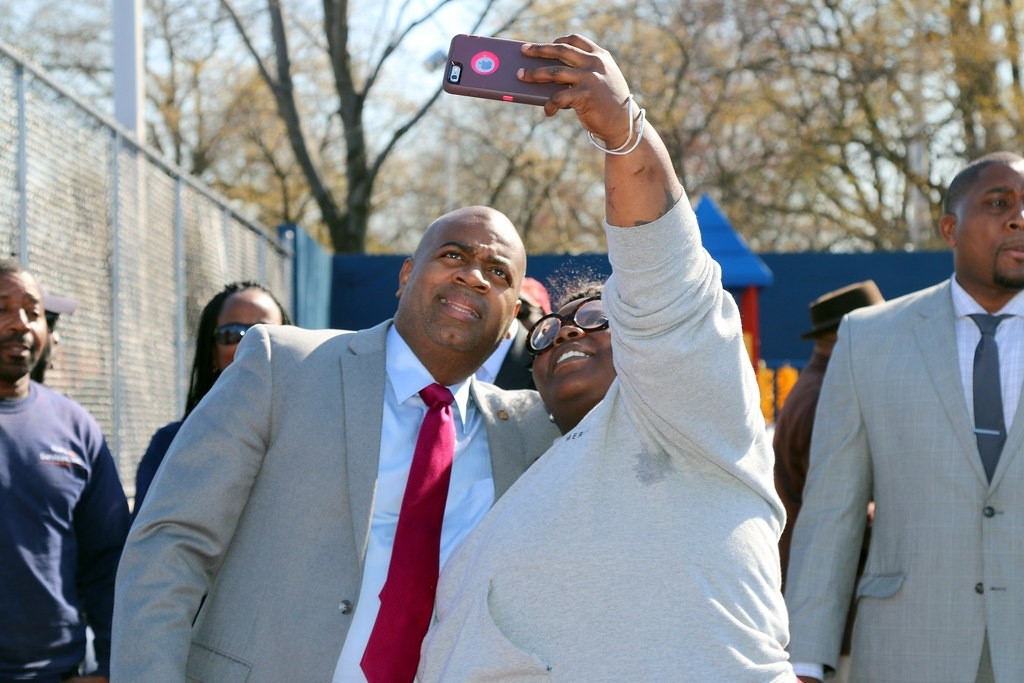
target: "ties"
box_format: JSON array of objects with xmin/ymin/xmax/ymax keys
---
[
  {"xmin": 357, "ymin": 383, "xmax": 457, "ymax": 683},
  {"xmin": 965, "ymin": 313, "xmax": 1012, "ymax": 483}
]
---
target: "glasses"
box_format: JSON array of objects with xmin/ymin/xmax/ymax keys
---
[
  {"xmin": 208, "ymin": 321, "xmax": 268, "ymax": 345},
  {"xmin": 524, "ymin": 295, "xmax": 610, "ymax": 355}
]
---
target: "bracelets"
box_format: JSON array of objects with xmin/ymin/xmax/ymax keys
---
[{"xmin": 588, "ymin": 93, "xmax": 646, "ymax": 155}]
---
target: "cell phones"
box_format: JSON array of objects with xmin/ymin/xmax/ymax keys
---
[{"xmin": 443, "ymin": 34, "xmax": 574, "ymax": 109}]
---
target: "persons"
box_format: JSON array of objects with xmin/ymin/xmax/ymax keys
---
[
  {"xmin": 412, "ymin": 34, "xmax": 801, "ymax": 683},
  {"xmin": 0, "ymin": 258, "xmax": 131, "ymax": 683},
  {"xmin": 108, "ymin": 204, "xmax": 567, "ymax": 683},
  {"xmin": 474, "ymin": 277, "xmax": 553, "ymax": 392},
  {"xmin": 784, "ymin": 151, "xmax": 1024, "ymax": 682},
  {"xmin": 771, "ymin": 279, "xmax": 888, "ymax": 683},
  {"xmin": 132, "ymin": 280, "xmax": 290, "ymax": 539}
]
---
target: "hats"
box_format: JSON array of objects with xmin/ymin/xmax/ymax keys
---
[
  {"xmin": 38, "ymin": 288, "xmax": 78, "ymax": 317},
  {"xmin": 800, "ymin": 279, "xmax": 886, "ymax": 338}
]
